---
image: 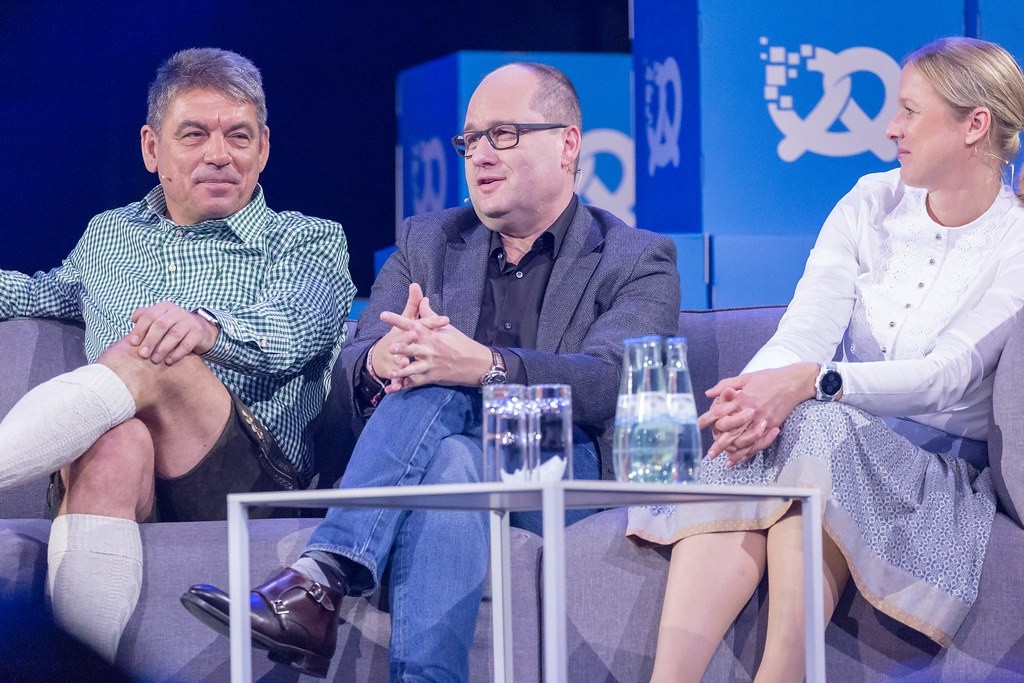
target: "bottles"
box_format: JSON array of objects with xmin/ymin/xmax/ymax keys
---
[{"xmin": 612, "ymin": 334, "xmax": 703, "ymax": 483}]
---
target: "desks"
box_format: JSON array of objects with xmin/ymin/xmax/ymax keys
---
[{"xmin": 225, "ymin": 478, "xmax": 826, "ymax": 683}]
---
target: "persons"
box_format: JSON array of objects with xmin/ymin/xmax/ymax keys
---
[
  {"xmin": 180, "ymin": 60, "xmax": 681, "ymax": 683},
  {"xmin": 1, "ymin": 48, "xmax": 360, "ymax": 661},
  {"xmin": 651, "ymin": 34, "xmax": 1024, "ymax": 683}
]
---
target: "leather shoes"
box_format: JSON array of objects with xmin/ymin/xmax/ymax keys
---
[{"xmin": 179, "ymin": 567, "xmax": 343, "ymax": 679}]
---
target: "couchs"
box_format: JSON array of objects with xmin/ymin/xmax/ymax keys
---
[{"xmin": 0, "ymin": 312, "xmax": 1024, "ymax": 683}]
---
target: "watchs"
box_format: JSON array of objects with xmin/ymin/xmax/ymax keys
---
[
  {"xmin": 813, "ymin": 361, "xmax": 843, "ymax": 402},
  {"xmin": 478, "ymin": 348, "xmax": 508, "ymax": 393}
]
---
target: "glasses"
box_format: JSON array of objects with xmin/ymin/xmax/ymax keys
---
[{"xmin": 451, "ymin": 124, "xmax": 568, "ymax": 157}]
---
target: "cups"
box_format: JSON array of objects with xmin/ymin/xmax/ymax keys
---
[
  {"xmin": 480, "ymin": 382, "xmax": 526, "ymax": 483},
  {"xmin": 527, "ymin": 384, "xmax": 575, "ymax": 481}
]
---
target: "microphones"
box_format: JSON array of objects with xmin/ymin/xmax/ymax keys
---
[
  {"xmin": 157, "ymin": 169, "xmax": 166, "ymax": 180},
  {"xmin": 464, "ymin": 197, "xmax": 470, "ymax": 203}
]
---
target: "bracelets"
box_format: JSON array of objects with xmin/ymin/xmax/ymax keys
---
[{"xmin": 193, "ymin": 307, "xmax": 221, "ymax": 333}]
